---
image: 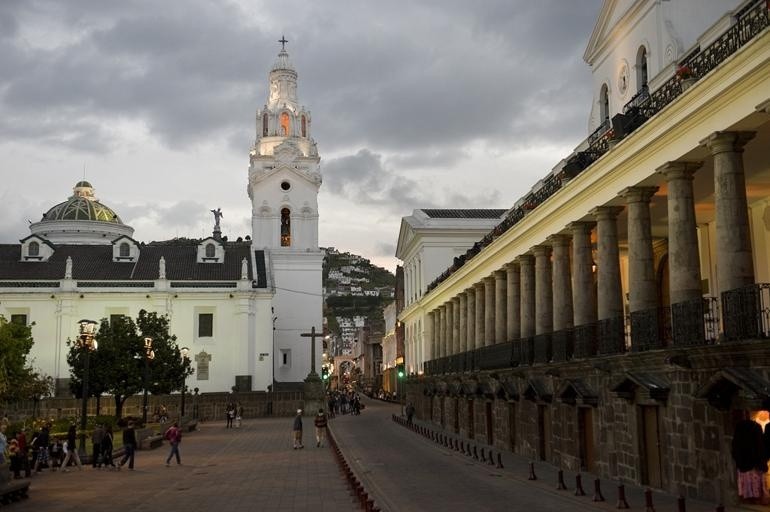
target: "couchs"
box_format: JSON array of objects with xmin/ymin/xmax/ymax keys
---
[
  {"xmin": 398, "ymin": 364, "xmax": 405, "ymax": 381},
  {"xmin": 321, "ymin": 366, "xmax": 328, "ymax": 380}
]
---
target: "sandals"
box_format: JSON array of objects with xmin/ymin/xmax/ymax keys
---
[{"xmin": 343, "ymin": 373, "xmax": 349, "ymax": 384}]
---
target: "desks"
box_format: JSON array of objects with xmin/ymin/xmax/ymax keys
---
[
  {"xmin": 72, "ymin": 315, "xmax": 100, "ymax": 461},
  {"xmin": 180, "ymin": 344, "xmax": 190, "ymax": 416},
  {"xmin": 135, "ymin": 333, "xmax": 155, "ymax": 425}
]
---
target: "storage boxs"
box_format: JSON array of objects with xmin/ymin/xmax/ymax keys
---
[
  {"xmin": 180, "ymin": 416, "xmax": 197, "ymax": 433},
  {"xmin": 0, "ymin": 464, "xmax": 31, "ymax": 504},
  {"xmin": 136, "ymin": 428, "xmax": 164, "ymax": 449}
]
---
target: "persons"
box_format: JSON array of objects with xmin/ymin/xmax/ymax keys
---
[
  {"xmin": 211, "ymin": 208, "xmax": 223, "ymax": 226},
  {"xmin": 266, "ymin": 385, "xmax": 274, "ymax": 417},
  {"xmin": 0, "ymin": 404, "xmax": 182, "ymax": 478},
  {"xmin": 406, "ymin": 402, "xmax": 415, "ymax": 426},
  {"xmin": 327, "ymin": 376, "xmax": 398, "ymax": 418},
  {"xmin": 315, "ymin": 409, "xmax": 327, "ymax": 449},
  {"xmin": 225, "ymin": 401, "xmax": 236, "ymax": 430},
  {"xmin": 236, "ymin": 401, "xmax": 243, "ymax": 428},
  {"xmin": 192, "ymin": 387, "xmax": 200, "ymax": 420},
  {"xmin": 293, "ymin": 408, "xmax": 304, "ymax": 449}
]
